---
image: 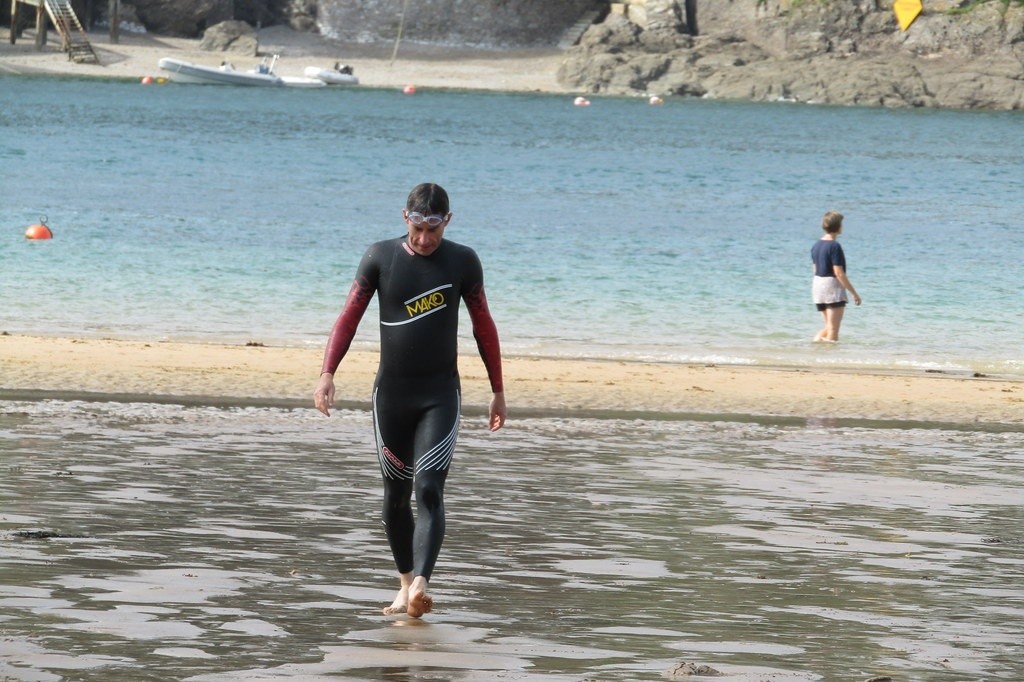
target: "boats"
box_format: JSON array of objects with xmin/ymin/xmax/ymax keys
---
[
  {"xmin": 158, "ymin": 58, "xmax": 327, "ymax": 88},
  {"xmin": 303, "ymin": 63, "xmax": 357, "ymax": 86}
]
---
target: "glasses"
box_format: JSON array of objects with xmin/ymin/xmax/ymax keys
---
[{"xmin": 406, "ymin": 211, "xmax": 449, "ymax": 225}]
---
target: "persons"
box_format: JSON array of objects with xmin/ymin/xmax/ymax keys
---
[
  {"xmin": 314, "ymin": 183, "xmax": 507, "ymax": 618},
  {"xmin": 811, "ymin": 211, "xmax": 861, "ymax": 340}
]
